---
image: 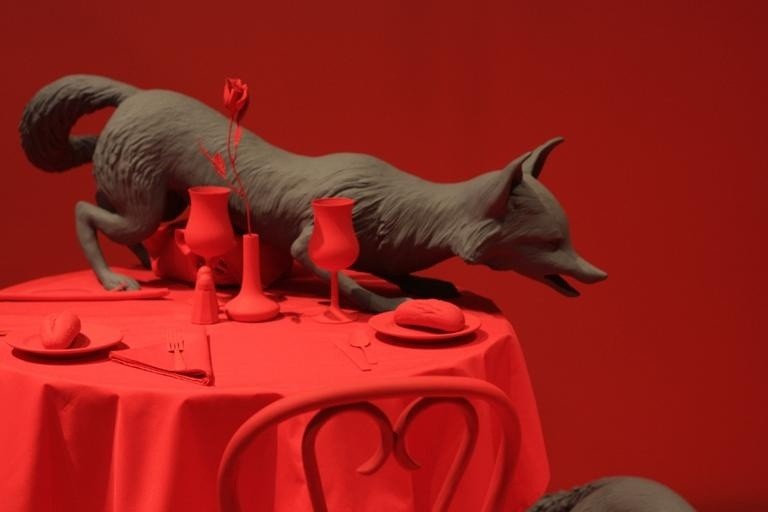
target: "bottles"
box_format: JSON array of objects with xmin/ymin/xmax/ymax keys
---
[{"xmin": 189, "ymin": 267, "xmax": 220, "ymax": 325}]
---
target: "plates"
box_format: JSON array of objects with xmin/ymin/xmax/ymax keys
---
[
  {"xmin": 4, "ymin": 324, "xmax": 123, "ymax": 359},
  {"xmin": 369, "ymin": 309, "xmax": 482, "ymax": 343}
]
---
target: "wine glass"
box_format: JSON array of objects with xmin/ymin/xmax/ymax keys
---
[
  {"xmin": 185, "ymin": 185, "xmax": 236, "ymax": 273},
  {"xmin": 307, "ymin": 198, "xmax": 360, "ymax": 322}
]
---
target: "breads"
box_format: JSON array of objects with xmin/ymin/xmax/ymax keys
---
[
  {"xmin": 41, "ymin": 309, "xmax": 82, "ymax": 350},
  {"xmin": 391, "ymin": 299, "xmax": 468, "ymax": 333}
]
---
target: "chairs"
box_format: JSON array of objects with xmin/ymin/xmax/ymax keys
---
[{"xmin": 213, "ymin": 374, "xmax": 523, "ymax": 512}]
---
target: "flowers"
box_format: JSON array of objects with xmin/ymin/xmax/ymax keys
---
[{"xmin": 201, "ymin": 77, "xmax": 253, "ymax": 236}]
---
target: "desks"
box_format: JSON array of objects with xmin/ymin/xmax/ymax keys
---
[{"xmin": 0, "ymin": 266, "xmax": 516, "ymax": 512}]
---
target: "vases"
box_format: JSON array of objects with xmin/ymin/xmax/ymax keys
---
[{"xmin": 223, "ymin": 232, "xmax": 280, "ymax": 323}]
